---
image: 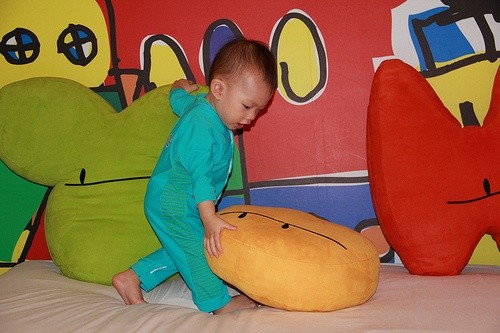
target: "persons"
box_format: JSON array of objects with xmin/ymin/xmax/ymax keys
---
[{"xmin": 111, "ymin": 36, "xmax": 278, "ymax": 316}]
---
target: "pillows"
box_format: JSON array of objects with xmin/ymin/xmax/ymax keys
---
[
  {"xmin": 0, "ymin": 77, "xmax": 209, "ymax": 285},
  {"xmin": 204, "ymin": 205, "xmax": 381, "ymax": 312},
  {"xmin": 366, "ymin": 59, "xmax": 500, "ymax": 276}
]
